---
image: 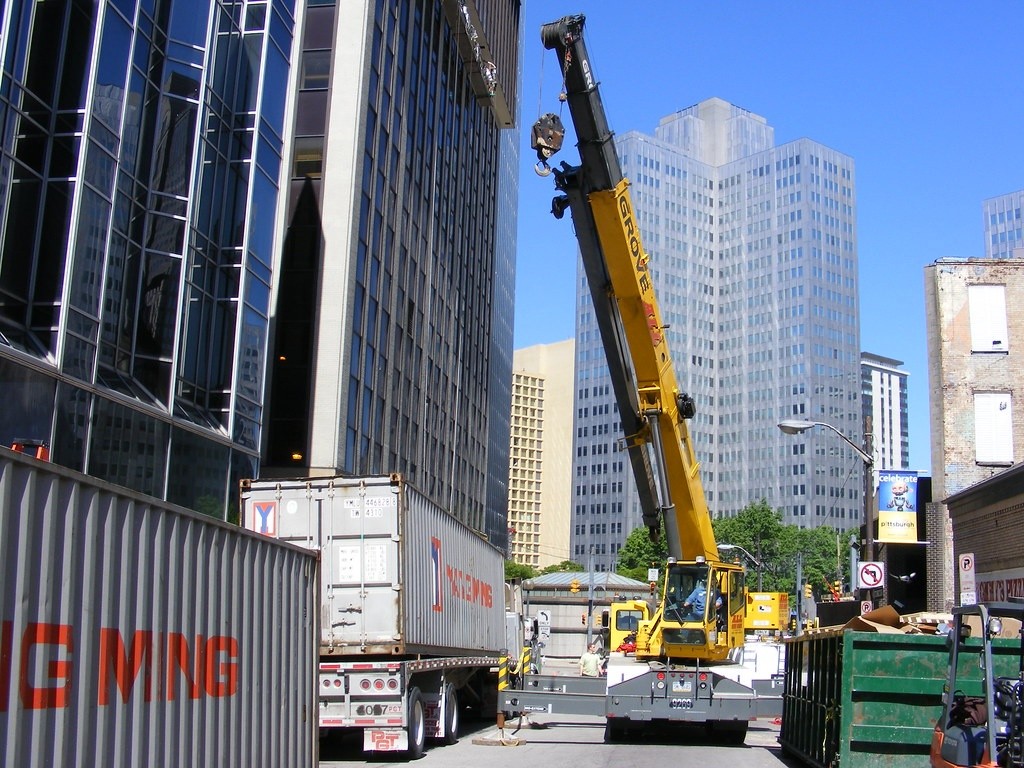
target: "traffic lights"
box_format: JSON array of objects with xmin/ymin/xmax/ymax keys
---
[
  {"xmin": 792, "ymin": 618, "xmax": 796, "ymax": 630},
  {"xmin": 805, "ymin": 583, "xmax": 812, "ymax": 597},
  {"xmin": 807, "ymin": 620, "xmax": 812, "ymax": 630},
  {"xmin": 569, "ymin": 578, "xmax": 581, "ymax": 596},
  {"xmin": 833, "ymin": 580, "xmax": 841, "ymax": 597},
  {"xmin": 596, "ymin": 615, "xmax": 602, "ymax": 625},
  {"xmin": 650, "ymin": 581, "xmax": 656, "ymax": 595},
  {"xmin": 581, "ymin": 613, "xmax": 587, "ymax": 624}
]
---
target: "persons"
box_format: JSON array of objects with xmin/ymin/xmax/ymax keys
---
[
  {"xmin": 580, "ymin": 643, "xmax": 603, "ymax": 678},
  {"xmin": 680, "ymin": 573, "xmax": 723, "ymax": 643}
]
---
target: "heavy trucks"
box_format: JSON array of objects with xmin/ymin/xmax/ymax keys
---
[{"xmin": 232, "ymin": 470, "xmax": 508, "ymax": 763}]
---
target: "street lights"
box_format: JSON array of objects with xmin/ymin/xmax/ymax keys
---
[
  {"xmin": 718, "ymin": 544, "xmax": 763, "ymax": 593},
  {"xmin": 778, "ymin": 414, "xmax": 880, "ymax": 613}
]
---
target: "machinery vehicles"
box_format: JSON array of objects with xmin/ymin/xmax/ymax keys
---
[
  {"xmin": 928, "ymin": 599, "xmax": 1023, "ymax": 768},
  {"xmin": 472, "ymin": 11, "xmax": 789, "ymax": 747}
]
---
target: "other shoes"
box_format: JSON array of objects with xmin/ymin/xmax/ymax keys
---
[
  {"xmin": 676, "ymin": 635, "xmax": 688, "ymax": 643},
  {"xmin": 692, "ymin": 639, "xmax": 701, "ymax": 644}
]
